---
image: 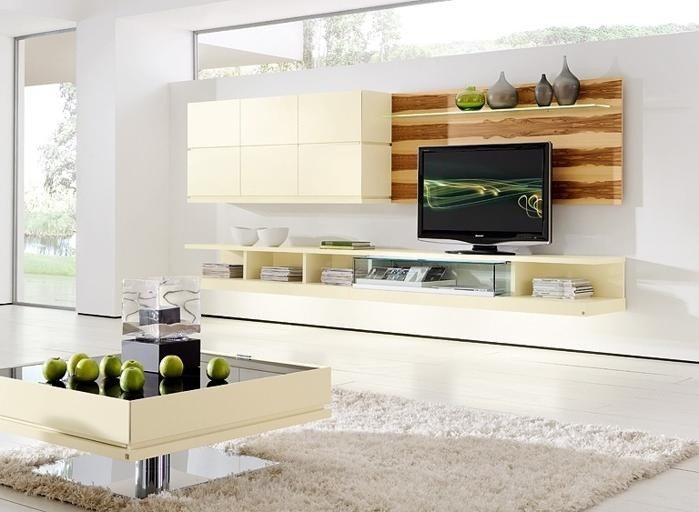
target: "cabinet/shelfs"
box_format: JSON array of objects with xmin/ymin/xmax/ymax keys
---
[
  {"xmin": 182, "ymin": 237, "xmax": 629, "ymax": 321},
  {"xmin": 185, "ymin": 91, "xmax": 394, "ymax": 207}
]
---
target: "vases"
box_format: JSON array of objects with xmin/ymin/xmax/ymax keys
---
[
  {"xmin": 532, "ymin": 71, "xmax": 555, "ymax": 106},
  {"xmin": 549, "ymin": 52, "xmax": 584, "ymax": 106},
  {"xmin": 456, "ymin": 84, "xmax": 486, "ymax": 111}
]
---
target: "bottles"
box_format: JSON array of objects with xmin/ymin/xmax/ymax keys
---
[
  {"xmin": 534, "ymin": 74, "xmax": 554, "ymax": 108},
  {"xmin": 454, "ymin": 86, "xmax": 484, "ymax": 111},
  {"xmin": 552, "ymin": 54, "xmax": 581, "ymax": 106}
]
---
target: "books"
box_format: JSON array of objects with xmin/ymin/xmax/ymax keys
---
[
  {"xmin": 259, "ymin": 265, "xmax": 302, "ymax": 281},
  {"xmin": 201, "ymin": 262, "xmax": 243, "ymax": 279},
  {"xmin": 530, "ymin": 276, "xmax": 594, "ymax": 298},
  {"xmin": 321, "ymin": 259, "xmax": 448, "ymax": 285}
]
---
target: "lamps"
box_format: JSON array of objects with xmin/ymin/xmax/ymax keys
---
[{"xmin": 117, "ymin": 271, "xmax": 205, "ymax": 379}]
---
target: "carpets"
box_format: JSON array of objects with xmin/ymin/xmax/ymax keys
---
[{"xmin": 0, "ymin": 384, "xmax": 699, "ymax": 512}]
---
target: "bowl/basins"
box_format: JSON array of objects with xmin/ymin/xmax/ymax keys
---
[
  {"xmin": 257, "ymin": 226, "xmax": 288, "ymax": 248},
  {"xmin": 231, "ymin": 225, "xmax": 267, "ymax": 247}
]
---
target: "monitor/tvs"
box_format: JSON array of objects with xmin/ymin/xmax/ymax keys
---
[{"xmin": 415, "ymin": 141, "xmax": 552, "ymax": 255}]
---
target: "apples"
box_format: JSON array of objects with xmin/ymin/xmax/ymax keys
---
[
  {"xmin": 159, "ymin": 377, "xmax": 184, "ymax": 395},
  {"xmin": 120, "ymin": 367, "xmax": 145, "ymax": 393},
  {"xmin": 75, "ymin": 358, "xmax": 99, "ymax": 382},
  {"xmin": 43, "ymin": 358, "xmax": 67, "ymax": 381},
  {"xmin": 206, "ymin": 357, "xmax": 230, "ymax": 379},
  {"xmin": 159, "ymin": 355, "xmax": 184, "ymax": 378},
  {"xmin": 120, "ymin": 361, "xmax": 144, "ymax": 376},
  {"xmin": 66, "ymin": 353, "xmax": 88, "ymax": 377},
  {"xmin": 99, "ymin": 356, "xmax": 122, "ymax": 377},
  {"xmin": 68, "ymin": 376, "xmax": 122, "ymax": 397}
]
